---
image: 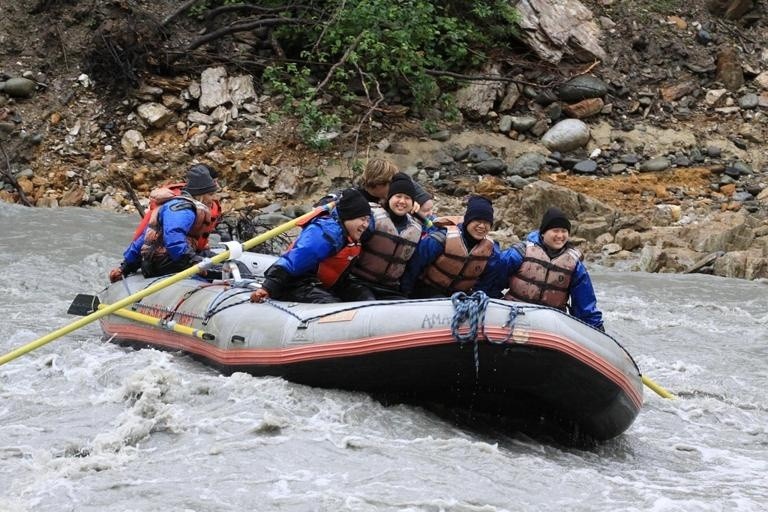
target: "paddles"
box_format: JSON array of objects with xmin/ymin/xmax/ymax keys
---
[{"xmin": 67, "ymin": 292, "xmax": 215, "ymax": 343}]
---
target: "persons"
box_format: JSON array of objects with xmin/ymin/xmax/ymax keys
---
[
  {"xmin": 109, "ymin": 163, "xmax": 222, "ymax": 282},
  {"xmin": 498, "ymin": 207, "xmax": 605, "ymax": 332},
  {"xmin": 359, "ymin": 159, "xmax": 397, "ymax": 203},
  {"xmin": 401, "ymin": 195, "xmax": 499, "ymax": 299},
  {"xmin": 339, "ymin": 173, "xmax": 422, "ymax": 302},
  {"xmin": 252, "ymin": 189, "xmax": 371, "ymax": 303},
  {"xmin": 413, "ymin": 179, "xmax": 439, "ymax": 233},
  {"xmin": 140, "ymin": 166, "xmax": 252, "ymax": 281}
]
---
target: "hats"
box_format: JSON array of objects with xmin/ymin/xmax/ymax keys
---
[
  {"xmin": 196, "ymin": 163, "xmax": 219, "ymax": 179},
  {"xmin": 386, "ymin": 172, "xmax": 417, "ymax": 201},
  {"xmin": 540, "ymin": 206, "xmax": 571, "ymax": 233},
  {"xmin": 338, "ymin": 188, "xmax": 372, "ymax": 219},
  {"xmin": 182, "ymin": 165, "xmax": 217, "ymax": 196},
  {"xmin": 411, "ymin": 179, "xmax": 434, "ymax": 206},
  {"xmin": 464, "ymin": 195, "xmax": 494, "ymax": 225}
]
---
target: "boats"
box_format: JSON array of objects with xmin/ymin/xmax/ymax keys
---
[{"xmin": 99, "ymin": 248, "xmax": 643, "ymax": 449}]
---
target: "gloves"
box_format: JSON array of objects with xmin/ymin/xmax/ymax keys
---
[
  {"xmin": 108, "ymin": 266, "xmax": 124, "ymax": 282},
  {"xmin": 249, "ymin": 287, "xmax": 268, "ymax": 304}
]
